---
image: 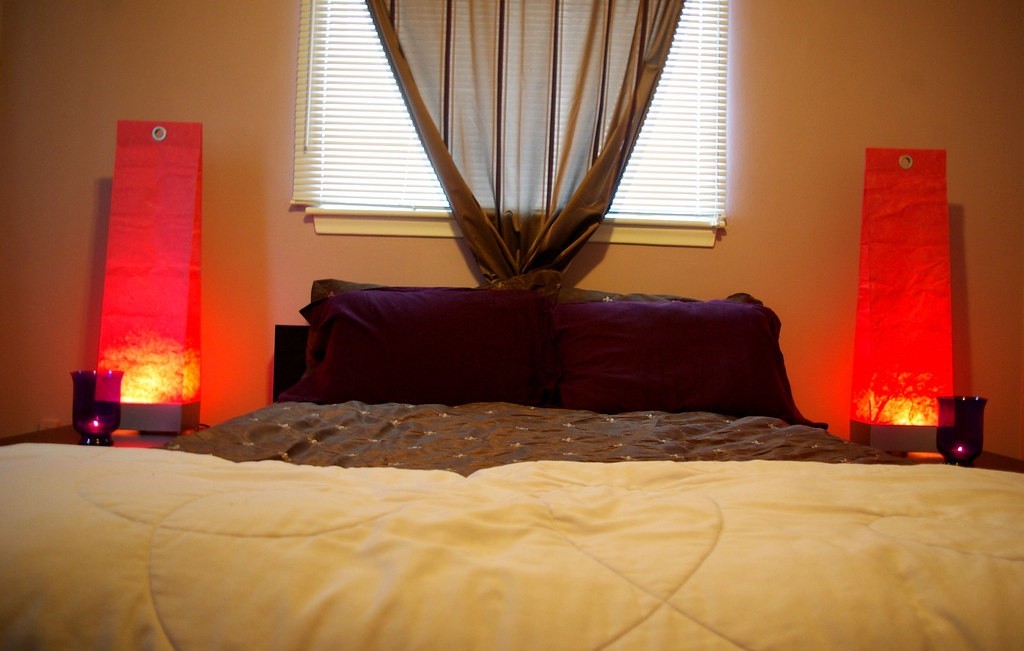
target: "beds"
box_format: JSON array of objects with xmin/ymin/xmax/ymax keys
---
[{"xmin": 0, "ymin": 280, "xmax": 1023, "ymax": 651}]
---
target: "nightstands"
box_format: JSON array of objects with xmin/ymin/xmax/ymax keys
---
[
  {"xmin": 912, "ymin": 449, "xmax": 1024, "ymax": 474},
  {"xmin": 0, "ymin": 424, "xmax": 198, "ymax": 449}
]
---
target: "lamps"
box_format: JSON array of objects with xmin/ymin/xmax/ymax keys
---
[
  {"xmin": 68, "ymin": 369, "xmax": 125, "ymax": 446},
  {"xmin": 94, "ymin": 119, "xmax": 205, "ymax": 434},
  {"xmin": 849, "ymin": 146, "xmax": 956, "ymax": 453},
  {"xmin": 937, "ymin": 395, "xmax": 984, "ymax": 466}
]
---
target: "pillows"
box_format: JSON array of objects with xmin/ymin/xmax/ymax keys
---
[
  {"xmin": 544, "ymin": 300, "xmax": 833, "ymax": 428},
  {"xmin": 277, "ymin": 284, "xmax": 565, "ymax": 410}
]
